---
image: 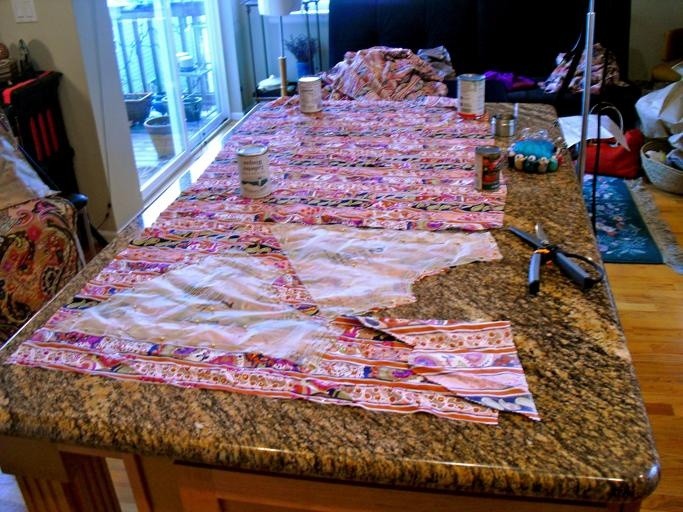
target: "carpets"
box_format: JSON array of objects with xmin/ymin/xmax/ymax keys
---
[{"xmin": 580, "ymin": 174, "xmax": 682, "ymax": 275}]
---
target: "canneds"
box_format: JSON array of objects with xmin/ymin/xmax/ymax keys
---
[
  {"xmin": 476, "ymin": 147, "xmax": 501, "ymax": 192},
  {"xmin": 298, "ymin": 75, "xmax": 323, "ymax": 114},
  {"xmin": 490, "ymin": 113, "xmax": 514, "ymax": 137},
  {"xmin": 457, "ymin": 74, "xmax": 486, "ymax": 117},
  {"xmin": 236, "ymin": 143, "xmax": 272, "ymax": 199}
]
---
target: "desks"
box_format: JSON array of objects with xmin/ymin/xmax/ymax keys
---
[
  {"xmin": 176, "ymin": 65, "xmax": 211, "ymax": 109},
  {"xmin": 0, "ymin": 101, "xmax": 661, "ymax": 512}
]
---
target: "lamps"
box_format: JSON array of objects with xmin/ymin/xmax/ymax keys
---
[{"xmin": 255, "ymin": 0, "xmax": 302, "ymax": 96}]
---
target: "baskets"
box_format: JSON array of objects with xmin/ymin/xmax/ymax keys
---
[{"xmin": 640, "ymin": 139, "xmax": 683, "ymax": 195}]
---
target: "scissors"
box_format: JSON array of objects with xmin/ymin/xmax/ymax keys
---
[{"xmin": 504, "ymin": 224, "xmax": 605, "ymax": 293}]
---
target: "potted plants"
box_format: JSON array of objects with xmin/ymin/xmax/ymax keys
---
[{"xmin": 281, "ymin": 31, "xmax": 321, "ymax": 78}]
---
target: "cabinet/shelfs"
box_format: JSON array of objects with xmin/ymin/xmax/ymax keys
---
[{"xmin": 0, "ymin": 68, "xmax": 78, "ymax": 202}]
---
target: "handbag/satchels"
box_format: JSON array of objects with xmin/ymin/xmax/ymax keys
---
[
  {"xmin": 537, "ymin": 42, "xmax": 630, "ymax": 97},
  {"xmin": 577, "ymin": 129, "xmax": 645, "ymax": 180}
]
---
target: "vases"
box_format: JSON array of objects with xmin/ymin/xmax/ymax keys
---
[
  {"xmin": 142, "ymin": 115, "xmax": 187, "ymax": 159},
  {"xmin": 123, "ymin": 92, "xmax": 153, "ymax": 120}
]
